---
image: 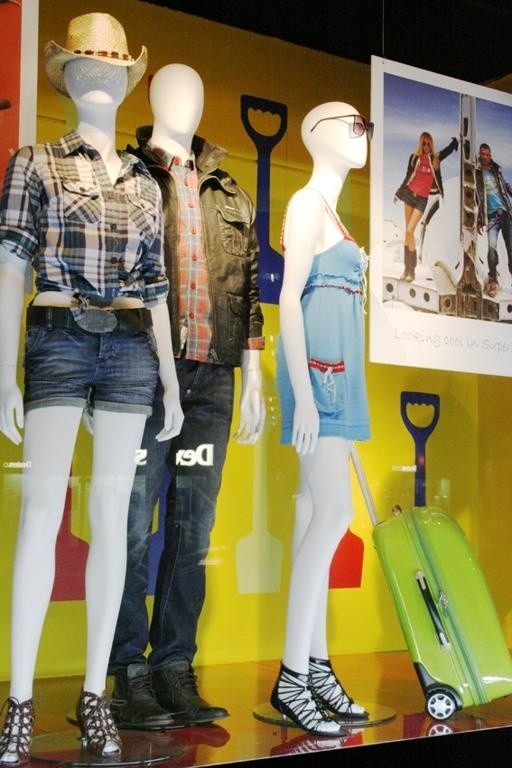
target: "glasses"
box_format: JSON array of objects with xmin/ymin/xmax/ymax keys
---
[{"xmin": 311, "ymin": 116, "xmax": 376, "ymax": 141}]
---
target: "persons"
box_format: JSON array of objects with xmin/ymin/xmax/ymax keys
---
[
  {"xmin": 393, "ymin": 132, "xmax": 460, "ymax": 282},
  {"xmin": 476, "ymin": 143, "xmax": 512, "ymax": 297},
  {"xmin": 270, "ymin": 102, "xmax": 374, "ymax": 737},
  {"xmin": 1, "ymin": 11, "xmax": 184, "ymax": 767},
  {"xmin": 77, "ymin": 62, "xmax": 267, "ymax": 730}
]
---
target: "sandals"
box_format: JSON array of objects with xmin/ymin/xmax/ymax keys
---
[
  {"xmin": 309, "ymin": 659, "xmax": 367, "ymax": 716},
  {"xmin": 271, "ymin": 663, "xmax": 347, "ymax": 736},
  {"xmin": 1, "ymin": 697, "xmax": 36, "ymax": 765},
  {"xmin": 78, "ymin": 688, "xmax": 122, "ymax": 756}
]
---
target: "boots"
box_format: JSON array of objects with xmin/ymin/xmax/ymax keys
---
[{"xmin": 400, "ymin": 245, "xmax": 417, "ymax": 283}]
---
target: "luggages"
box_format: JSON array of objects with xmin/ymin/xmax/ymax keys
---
[{"xmin": 374, "ymin": 502, "xmax": 511, "ymax": 706}]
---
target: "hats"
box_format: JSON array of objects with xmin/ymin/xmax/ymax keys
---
[{"xmin": 44, "ymin": 13, "xmax": 148, "ymax": 99}]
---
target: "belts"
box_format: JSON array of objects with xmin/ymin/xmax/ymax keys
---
[{"xmin": 26, "ymin": 303, "xmax": 153, "ymax": 339}]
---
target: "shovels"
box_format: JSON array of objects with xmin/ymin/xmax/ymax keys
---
[{"xmin": 238, "ymin": 92, "xmax": 288, "ymax": 305}]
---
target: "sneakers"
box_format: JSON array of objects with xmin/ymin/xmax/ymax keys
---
[
  {"xmin": 109, "ymin": 664, "xmax": 176, "ymax": 731},
  {"xmin": 487, "ymin": 276, "xmax": 496, "ymax": 297},
  {"xmin": 150, "ymin": 662, "xmax": 229, "ymax": 724}
]
